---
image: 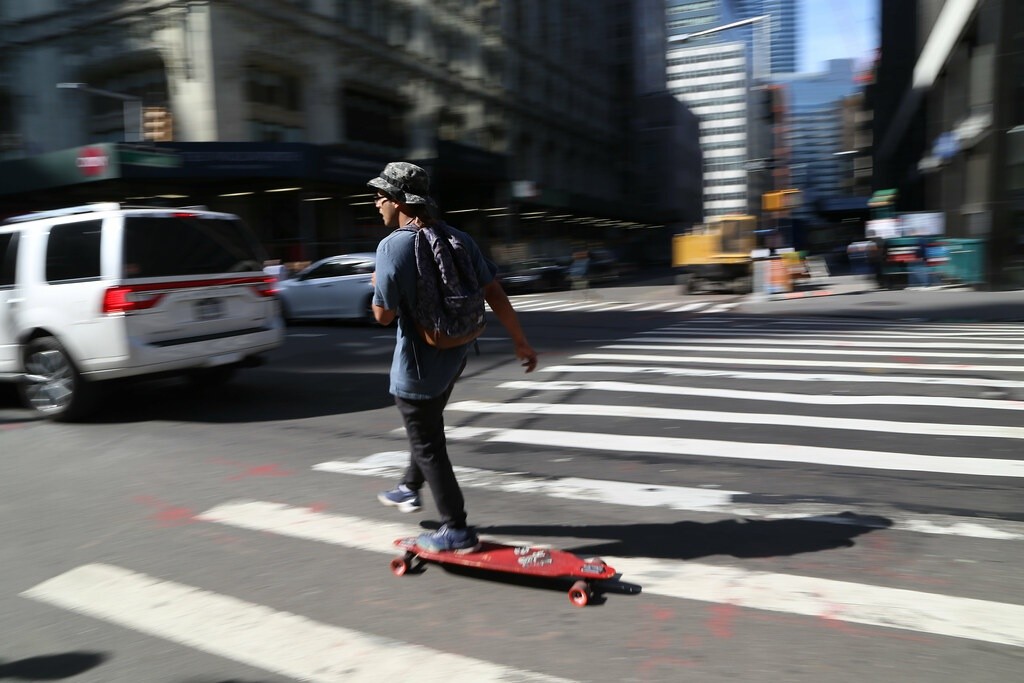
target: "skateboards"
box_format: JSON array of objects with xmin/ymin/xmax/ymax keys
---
[{"xmin": 390, "ymin": 536, "xmax": 615, "ymax": 608}]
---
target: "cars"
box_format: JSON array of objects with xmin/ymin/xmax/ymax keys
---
[{"xmin": 266, "ymin": 251, "xmax": 398, "ymax": 323}]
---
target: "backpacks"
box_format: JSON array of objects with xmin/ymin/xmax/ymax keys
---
[{"xmin": 390, "ymin": 214, "xmax": 489, "ymax": 349}]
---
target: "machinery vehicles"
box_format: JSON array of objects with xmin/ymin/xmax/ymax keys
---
[{"xmin": 670, "ymin": 213, "xmax": 760, "ymax": 297}]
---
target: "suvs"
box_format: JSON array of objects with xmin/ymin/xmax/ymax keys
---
[{"xmin": 0, "ymin": 190, "xmax": 286, "ymax": 422}]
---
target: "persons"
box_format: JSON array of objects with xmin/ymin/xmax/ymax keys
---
[{"xmin": 366, "ymin": 162, "xmax": 537, "ymax": 555}]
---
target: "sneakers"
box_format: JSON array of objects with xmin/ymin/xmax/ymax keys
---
[
  {"xmin": 377, "ymin": 484, "xmax": 423, "ymax": 513},
  {"xmin": 415, "ymin": 524, "xmax": 482, "ymax": 555}
]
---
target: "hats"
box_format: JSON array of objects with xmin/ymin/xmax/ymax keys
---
[{"xmin": 367, "ymin": 161, "xmax": 439, "ymax": 208}]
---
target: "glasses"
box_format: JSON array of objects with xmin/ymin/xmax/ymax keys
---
[{"xmin": 372, "ymin": 194, "xmax": 388, "ymax": 202}]
